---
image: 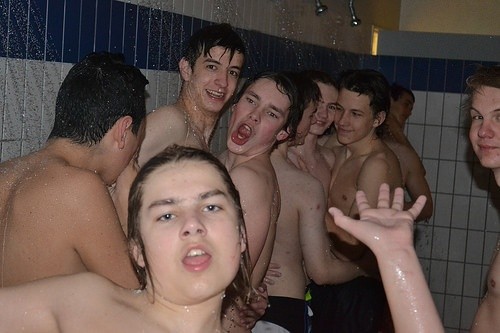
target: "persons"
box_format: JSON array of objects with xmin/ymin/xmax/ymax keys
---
[
  {"xmin": 0, "ymin": 144, "xmax": 446, "ymax": 333},
  {"xmin": 457, "ymin": 64, "xmax": 500, "ymax": 333},
  {"xmin": 105, "ymin": 23, "xmax": 434, "ymax": 333},
  {"xmin": 0, "ymin": 52, "xmax": 150, "ymax": 290}
]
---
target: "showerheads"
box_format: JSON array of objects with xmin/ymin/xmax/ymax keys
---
[
  {"xmin": 350, "ymin": 15, "xmax": 362, "ymax": 28},
  {"xmin": 315, "ymin": 2, "xmax": 329, "ymax": 17}
]
